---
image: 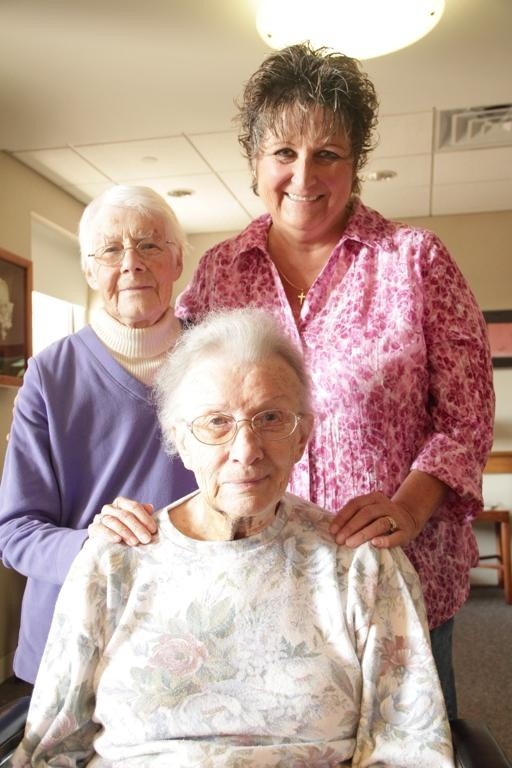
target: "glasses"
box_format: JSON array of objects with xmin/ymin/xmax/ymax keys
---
[
  {"xmin": 188, "ymin": 408, "xmax": 299, "ymax": 446},
  {"xmin": 87, "ymin": 238, "xmax": 176, "ymax": 267}
]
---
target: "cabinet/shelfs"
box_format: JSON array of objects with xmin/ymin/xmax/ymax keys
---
[{"xmin": 0, "ymin": 246, "xmax": 33, "ymax": 389}]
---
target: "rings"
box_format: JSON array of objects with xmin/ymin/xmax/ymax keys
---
[
  {"xmin": 385, "ymin": 517, "xmax": 399, "ymax": 532},
  {"xmin": 99, "ymin": 512, "xmax": 111, "ymax": 523}
]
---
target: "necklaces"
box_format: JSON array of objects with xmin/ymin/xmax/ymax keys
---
[{"xmin": 272, "ymin": 258, "xmax": 311, "ymax": 306}]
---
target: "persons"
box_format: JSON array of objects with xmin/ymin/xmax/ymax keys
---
[
  {"xmin": 0, "ymin": 303, "xmax": 462, "ymax": 768},
  {"xmin": 0, "ymin": 181, "xmax": 201, "ymax": 702},
  {"xmin": 171, "ymin": 40, "xmax": 499, "ymax": 728}
]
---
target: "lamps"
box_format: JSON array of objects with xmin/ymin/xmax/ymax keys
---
[{"xmin": 255, "ymin": 0, "xmax": 446, "ymax": 62}]
